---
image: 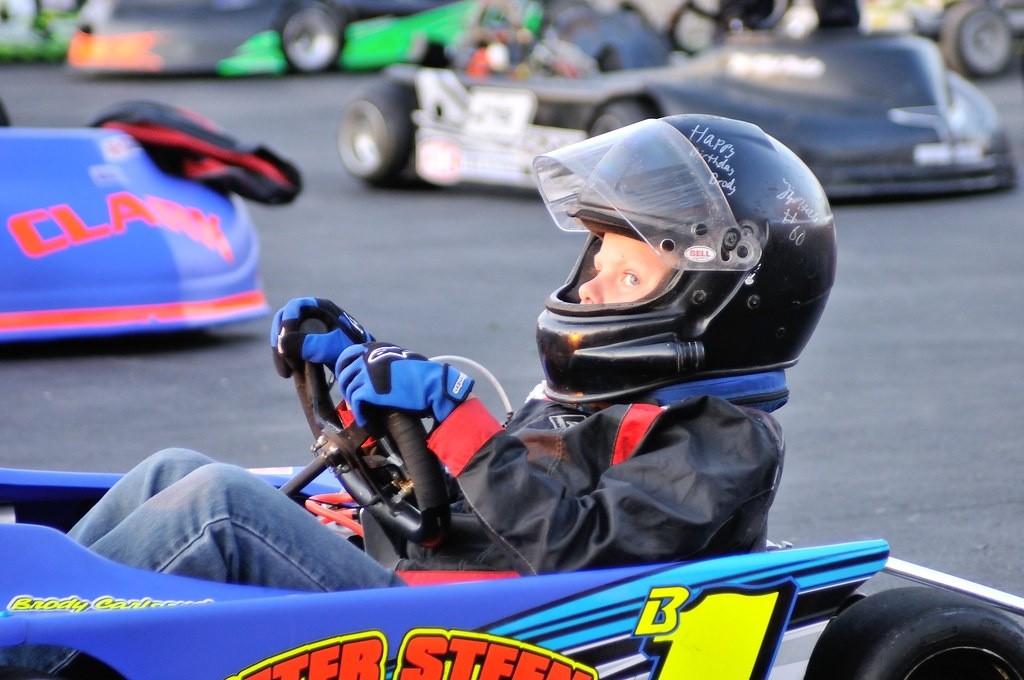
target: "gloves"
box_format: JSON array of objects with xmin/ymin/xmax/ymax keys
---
[
  {"xmin": 270, "ymin": 296, "xmax": 377, "ymax": 379},
  {"xmin": 335, "ymin": 341, "xmax": 475, "ymax": 427}
]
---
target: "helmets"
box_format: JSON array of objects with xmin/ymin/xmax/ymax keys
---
[{"xmin": 535, "ymin": 112, "xmax": 838, "ymax": 403}]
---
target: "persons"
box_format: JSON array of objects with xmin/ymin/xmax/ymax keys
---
[
  {"xmin": 717, "ymin": 0, "xmax": 859, "ymax": 32},
  {"xmin": 63, "ymin": 114, "xmax": 837, "ymax": 588}
]
